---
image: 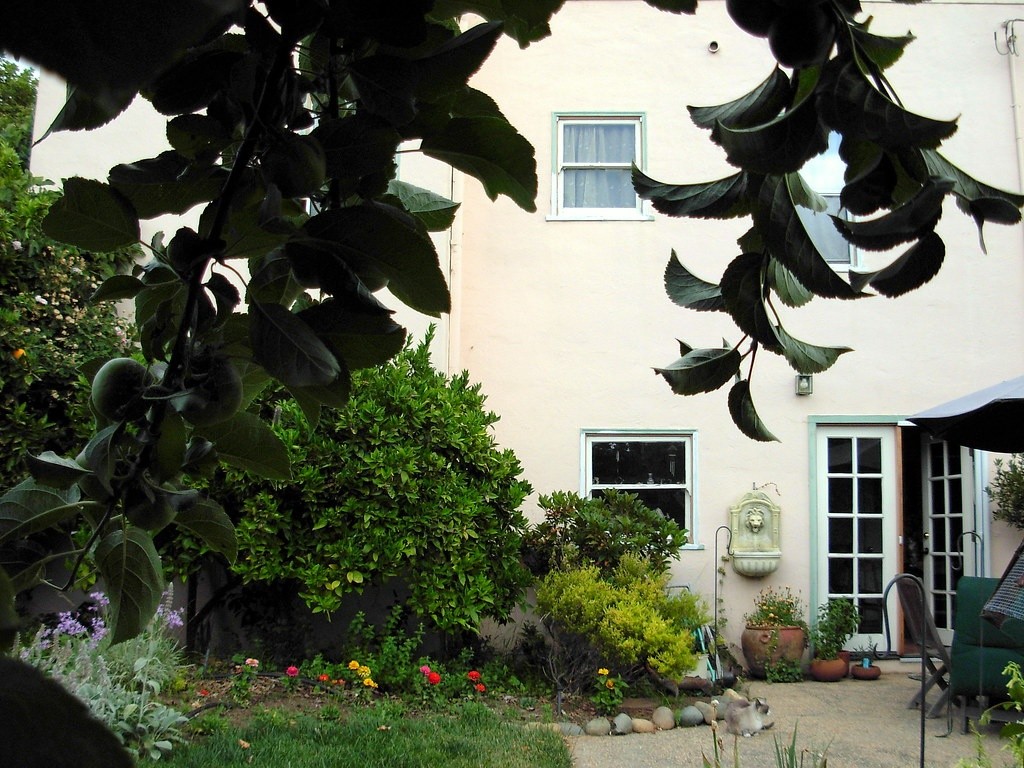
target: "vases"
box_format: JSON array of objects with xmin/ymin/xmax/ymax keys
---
[{"xmin": 740, "ymin": 625, "xmax": 806, "ymax": 679}]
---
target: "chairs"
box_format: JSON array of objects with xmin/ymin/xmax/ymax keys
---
[{"xmin": 894, "ymin": 573, "xmax": 1024, "ymax": 735}]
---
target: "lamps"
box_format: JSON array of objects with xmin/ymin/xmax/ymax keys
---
[{"xmin": 794, "ymin": 374, "xmax": 814, "ymax": 396}]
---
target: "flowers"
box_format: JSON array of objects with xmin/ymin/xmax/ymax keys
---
[{"xmin": 740, "ymin": 584, "xmax": 812, "ymax": 685}]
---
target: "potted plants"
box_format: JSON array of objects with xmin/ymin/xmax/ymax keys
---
[{"xmin": 807, "ymin": 594, "xmax": 882, "ymax": 682}]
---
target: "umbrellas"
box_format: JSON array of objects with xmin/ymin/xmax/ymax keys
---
[{"xmin": 905, "ymin": 376, "xmax": 1024, "ymax": 453}]
